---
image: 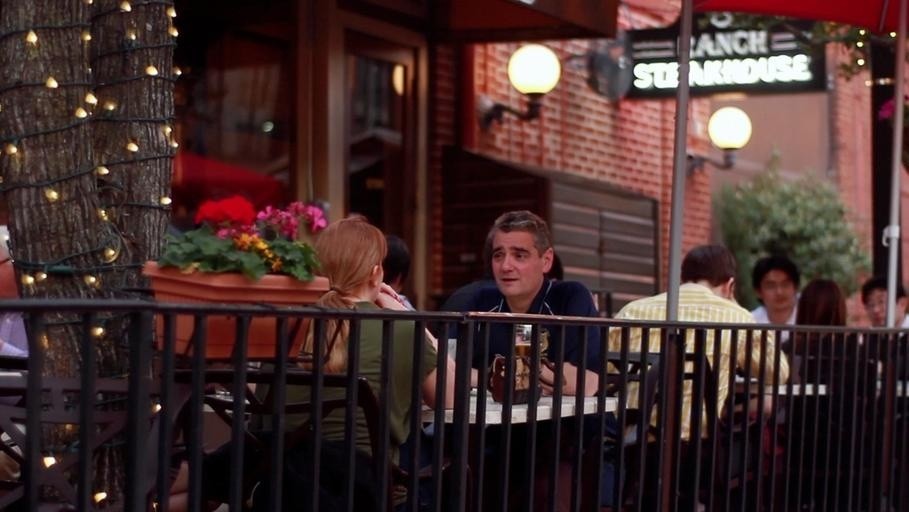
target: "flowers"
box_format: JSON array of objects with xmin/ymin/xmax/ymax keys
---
[{"xmin": 159, "ymin": 193, "xmax": 329, "ymax": 285}]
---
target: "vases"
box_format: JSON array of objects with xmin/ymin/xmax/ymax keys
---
[{"xmin": 143, "ymin": 262, "xmax": 331, "ymax": 361}]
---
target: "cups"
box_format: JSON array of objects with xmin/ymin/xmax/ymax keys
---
[{"xmin": 513, "ymin": 324, "xmax": 534, "ymax": 356}]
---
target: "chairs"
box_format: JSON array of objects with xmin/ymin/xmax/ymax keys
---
[{"xmin": 0, "ymin": 351, "xmax": 909, "ymax": 511}]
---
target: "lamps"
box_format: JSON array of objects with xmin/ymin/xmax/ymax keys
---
[
  {"xmin": 479, "ymin": 43, "xmax": 563, "ymax": 139},
  {"xmin": 682, "ymin": 106, "xmax": 753, "ymax": 189}
]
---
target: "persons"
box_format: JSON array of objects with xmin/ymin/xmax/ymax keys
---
[
  {"xmin": 749, "ymin": 257, "xmax": 800, "ymax": 336},
  {"xmin": 250, "ymin": 218, "xmax": 455, "ymax": 489},
  {"xmin": 782, "ymin": 279, "xmax": 859, "ymax": 384},
  {"xmin": 380, "ymin": 234, "xmax": 413, "ymax": 310},
  {"xmin": 860, "ymin": 275, "xmax": 909, "ymax": 329},
  {"xmin": 430, "ymin": 211, "xmax": 615, "ymax": 512},
  {"xmin": 607, "ymin": 244, "xmax": 788, "ymax": 494}
]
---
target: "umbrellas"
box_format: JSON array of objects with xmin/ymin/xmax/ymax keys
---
[{"xmin": 692, "ymin": 1, "xmax": 908, "ymax": 325}]
